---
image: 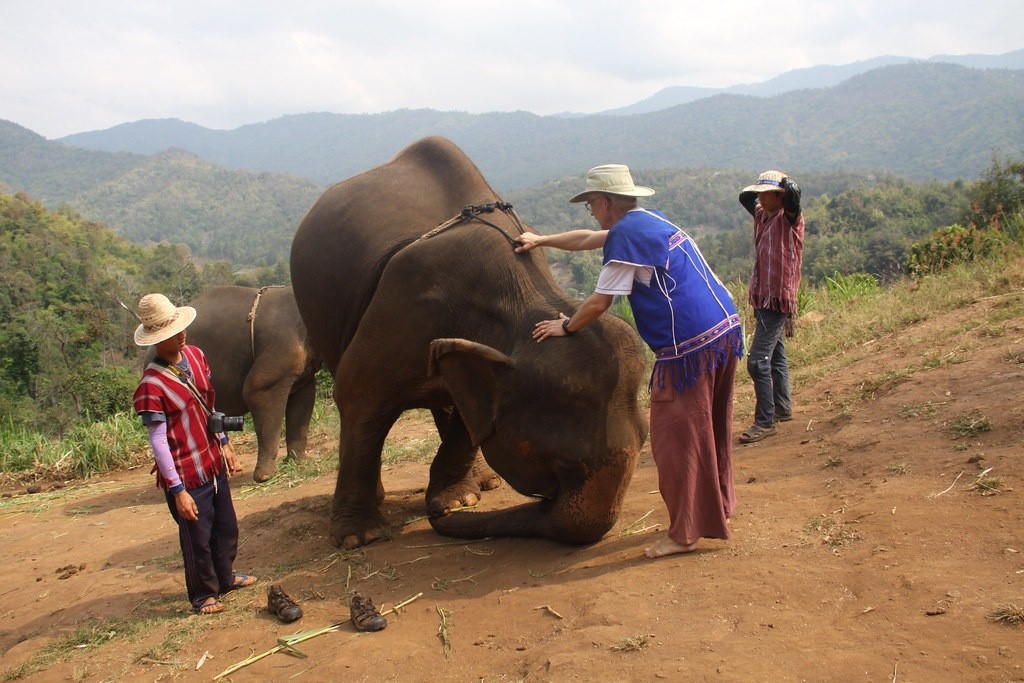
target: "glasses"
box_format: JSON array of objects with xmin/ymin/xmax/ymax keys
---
[{"xmin": 585, "ymin": 198, "xmax": 600, "ymax": 210}]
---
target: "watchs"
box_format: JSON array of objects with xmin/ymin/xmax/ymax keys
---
[{"xmin": 562, "ymin": 320, "xmax": 577, "ymax": 336}]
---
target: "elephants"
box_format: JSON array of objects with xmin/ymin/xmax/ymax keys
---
[
  {"xmin": 142, "ymin": 284, "xmax": 323, "ymax": 482},
  {"xmin": 288, "ymin": 134, "xmax": 647, "ymax": 551}
]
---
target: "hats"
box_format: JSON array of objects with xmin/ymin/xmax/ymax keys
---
[
  {"xmin": 134, "ymin": 293, "xmax": 196, "ymax": 346},
  {"xmin": 742, "ymin": 171, "xmax": 785, "ymax": 192},
  {"xmin": 568, "ymin": 164, "xmax": 655, "ymax": 203}
]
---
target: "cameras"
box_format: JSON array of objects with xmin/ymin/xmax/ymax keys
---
[{"xmin": 208, "ymin": 411, "xmax": 245, "ymax": 433}]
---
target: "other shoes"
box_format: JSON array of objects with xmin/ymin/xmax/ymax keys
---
[
  {"xmin": 350, "ymin": 594, "xmax": 387, "ymax": 632},
  {"xmin": 267, "ymin": 585, "xmax": 302, "ymax": 622}
]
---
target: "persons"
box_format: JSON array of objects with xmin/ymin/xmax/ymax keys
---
[
  {"xmin": 133, "ymin": 293, "xmax": 258, "ymax": 614},
  {"xmin": 513, "ymin": 165, "xmax": 745, "ymax": 559},
  {"xmin": 739, "ymin": 170, "xmax": 805, "ymax": 443}
]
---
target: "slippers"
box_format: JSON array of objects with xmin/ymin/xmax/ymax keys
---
[
  {"xmin": 738, "ymin": 426, "xmax": 777, "ymax": 442},
  {"xmin": 231, "ymin": 575, "xmax": 250, "ymax": 588},
  {"xmin": 192, "ymin": 599, "xmax": 223, "ymax": 615},
  {"xmin": 773, "ymin": 414, "xmax": 793, "ymax": 422}
]
